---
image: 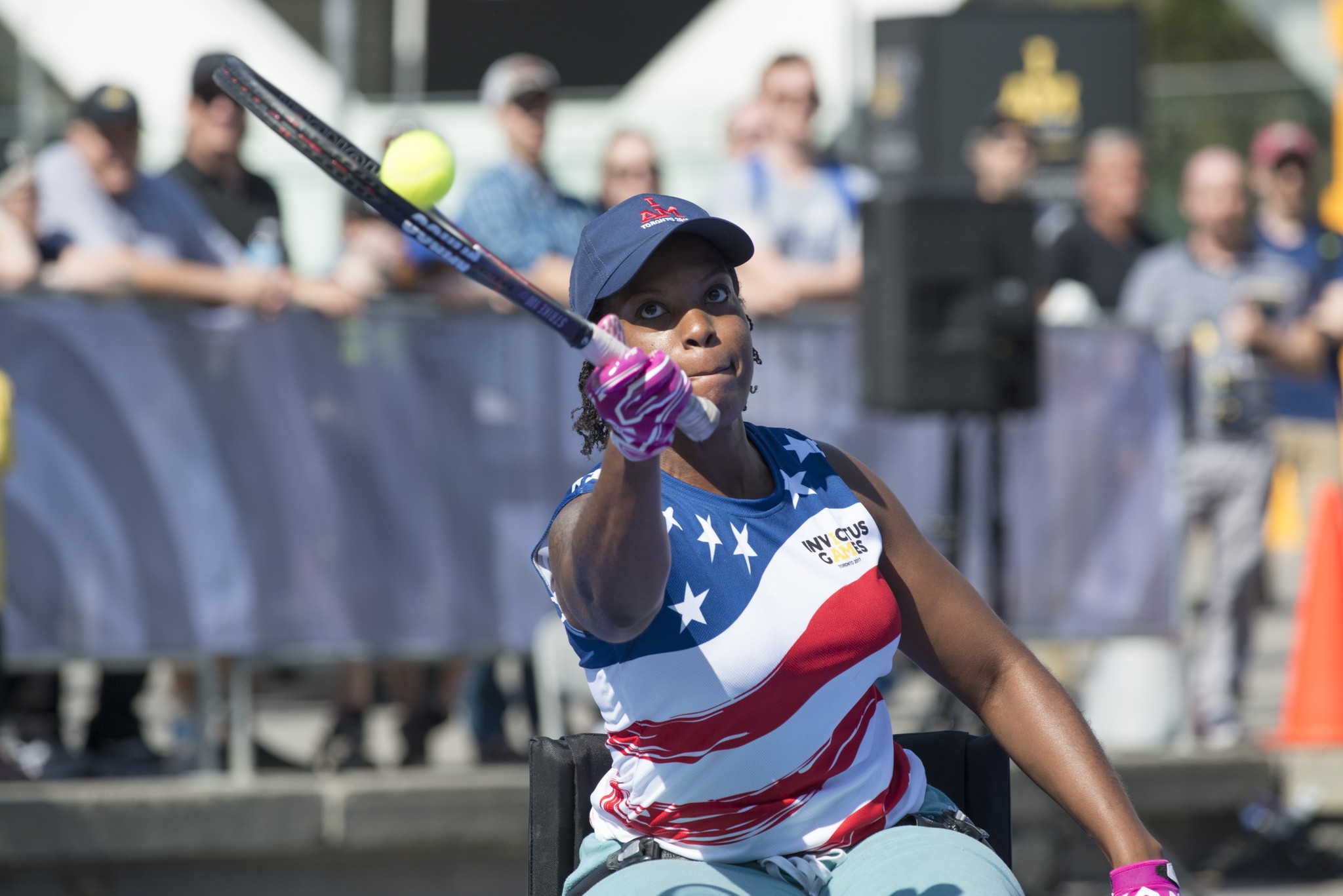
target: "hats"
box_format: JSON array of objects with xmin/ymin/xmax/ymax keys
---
[
  {"xmin": 73, "ymin": 87, "xmax": 140, "ymax": 129},
  {"xmin": 569, "ymin": 193, "xmax": 754, "ymax": 320}
]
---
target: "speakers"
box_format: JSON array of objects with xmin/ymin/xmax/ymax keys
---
[{"xmin": 854, "ymin": 197, "xmax": 1034, "ymax": 412}]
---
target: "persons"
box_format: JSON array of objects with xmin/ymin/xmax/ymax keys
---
[
  {"xmin": 530, "ymin": 193, "xmax": 1181, "ymax": 895},
  {"xmin": 0, "ymin": 54, "xmax": 1342, "ymax": 781}
]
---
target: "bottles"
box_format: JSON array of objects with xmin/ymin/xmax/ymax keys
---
[{"xmin": 243, "ymin": 218, "xmax": 282, "ymax": 268}]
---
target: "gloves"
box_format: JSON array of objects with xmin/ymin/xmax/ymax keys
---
[
  {"xmin": 1109, "ymin": 859, "xmax": 1180, "ymax": 896},
  {"xmin": 586, "ymin": 314, "xmax": 691, "ymax": 461}
]
